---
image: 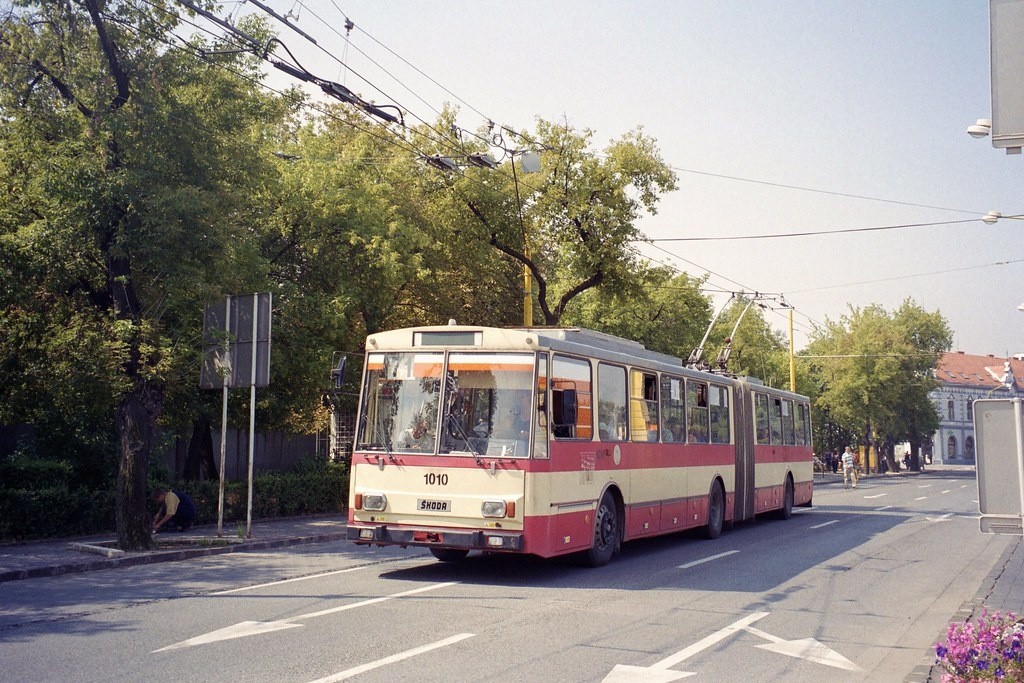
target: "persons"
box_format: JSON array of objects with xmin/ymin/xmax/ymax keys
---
[
  {"xmin": 646, "ymin": 413, "xmax": 672, "ymax": 442},
  {"xmin": 903, "ymin": 451, "xmax": 911, "ymax": 470},
  {"xmin": 669, "ymin": 422, "xmax": 685, "ymax": 444},
  {"xmin": 493, "ymin": 389, "xmax": 531, "ymax": 440},
  {"xmin": 842, "ymin": 446, "xmax": 858, "ymax": 488},
  {"xmin": 398, "ymin": 412, "xmax": 435, "ymax": 450},
  {"xmin": 824, "ymin": 448, "xmax": 831, "ymax": 473},
  {"xmin": 151, "ymin": 487, "xmax": 194, "ymax": 532},
  {"xmin": 832, "ymin": 448, "xmax": 839, "ymax": 473}
]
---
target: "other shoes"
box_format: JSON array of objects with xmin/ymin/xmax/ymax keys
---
[{"xmin": 168, "ymin": 523, "xmax": 191, "ymax": 533}]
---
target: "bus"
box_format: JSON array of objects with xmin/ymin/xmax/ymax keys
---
[{"xmin": 345, "ymin": 318, "xmax": 816, "ymax": 570}]
